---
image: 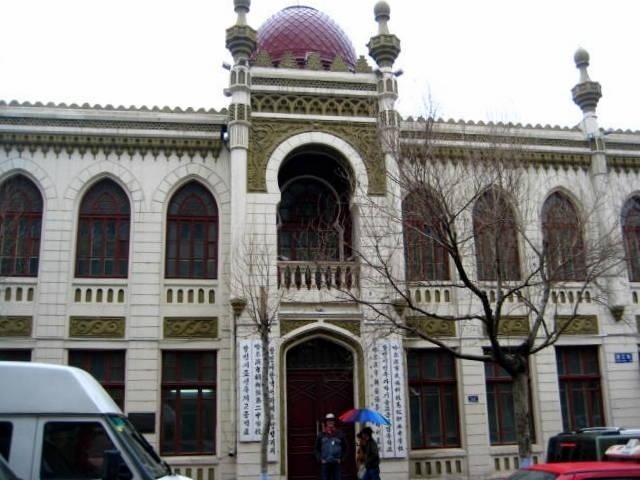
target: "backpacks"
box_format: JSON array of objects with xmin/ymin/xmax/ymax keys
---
[{"xmin": 321, "ymin": 430, "xmax": 343, "ymax": 464}]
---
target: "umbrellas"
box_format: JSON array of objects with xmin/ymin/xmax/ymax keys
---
[{"xmin": 337, "ymin": 407, "xmax": 391, "ymax": 430}]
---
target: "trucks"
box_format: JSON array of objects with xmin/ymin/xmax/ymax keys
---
[{"xmin": 0, "ymin": 359, "xmax": 193, "ymax": 480}]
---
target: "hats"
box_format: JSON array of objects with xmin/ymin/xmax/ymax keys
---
[{"xmin": 324, "ymin": 412, "xmax": 336, "ymax": 422}]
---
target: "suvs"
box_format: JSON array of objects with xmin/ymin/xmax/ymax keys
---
[{"xmin": 546, "ymin": 425, "xmax": 640, "ymax": 463}]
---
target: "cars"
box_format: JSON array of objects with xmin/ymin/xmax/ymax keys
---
[{"xmin": 501, "ymin": 460, "xmax": 639, "ymax": 480}]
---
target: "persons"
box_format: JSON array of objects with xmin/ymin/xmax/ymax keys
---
[
  {"xmin": 312, "ymin": 411, "xmax": 348, "ymax": 480},
  {"xmin": 359, "ymin": 427, "xmax": 382, "ymax": 480},
  {"xmin": 355, "ymin": 430, "xmax": 366, "ymax": 478}
]
